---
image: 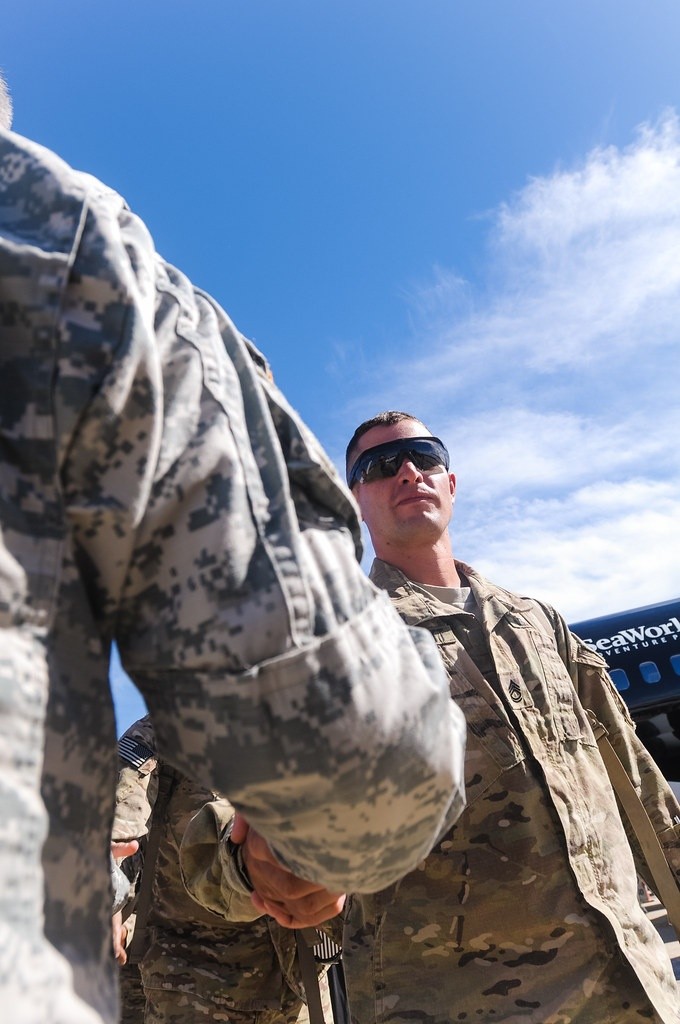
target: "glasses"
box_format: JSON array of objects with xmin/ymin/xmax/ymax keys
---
[{"xmin": 348, "ymin": 436, "xmax": 450, "ymax": 491}]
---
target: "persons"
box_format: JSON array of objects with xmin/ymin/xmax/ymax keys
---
[
  {"xmin": 0, "ymin": 73, "xmax": 466, "ymax": 1024},
  {"xmin": 111, "ymin": 713, "xmax": 340, "ymax": 1024},
  {"xmin": 178, "ymin": 411, "xmax": 680, "ymax": 1024}
]
